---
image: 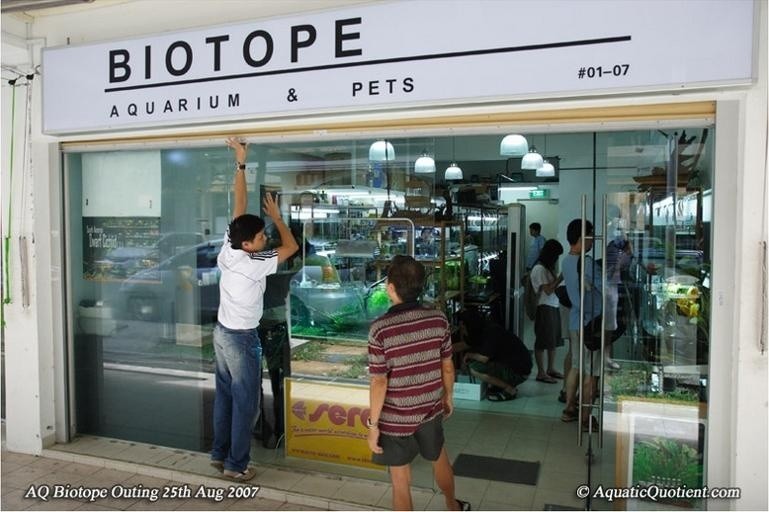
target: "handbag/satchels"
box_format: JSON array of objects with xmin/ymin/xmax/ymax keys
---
[
  {"xmin": 584, "ymin": 310, "xmax": 626, "ymax": 352},
  {"xmin": 555, "ymin": 285, "xmax": 572, "ymax": 308}
]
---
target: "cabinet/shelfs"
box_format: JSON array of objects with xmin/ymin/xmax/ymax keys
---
[
  {"xmin": 290, "ymin": 204, "xmax": 507, "ymax": 368},
  {"xmin": 81, "ymin": 149, "xmax": 176, "ymax": 285}
]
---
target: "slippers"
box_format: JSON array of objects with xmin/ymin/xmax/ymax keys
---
[
  {"xmin": 210, "ymin": 460, "xmax": 224, "ymax": 473},
  {"xmin": 224, "ymin": 467, "xmax": 256, "ymax": 481}
]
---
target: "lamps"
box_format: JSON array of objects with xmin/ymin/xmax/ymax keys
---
[
  {"xmin": 535, "ymin": 145, "xmax": 554, "ymax": 178},
  {"xmin": 502, "ymin": 135, "xmax": 527, "ymax": 157},
  {"xmin": 445, "ymin": 137, "xmax": 462, "ymax": 180},
  {"xmin": 413, "ymin": 142, "xmax": 436, "ymax": 173},
  {"xmin": 368, "ymin": 139, "xmax": 398, "ymax": 162},
  {"xmin": 521, "ymin": 139, "xmax": 542, "ymax": 169}
]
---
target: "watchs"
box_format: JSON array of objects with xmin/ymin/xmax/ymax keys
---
[
  {"xmin": 365, "ymin": 415, "xmax": 381, "ymax": 430},
  {"xmin": 231, "ymin": 160, "xmax": 248, "ymax": 175}
]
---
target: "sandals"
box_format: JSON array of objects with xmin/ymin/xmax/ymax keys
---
[{"xmin": 487, "ymin": 373, "xmax": 600, "ymax": 433}]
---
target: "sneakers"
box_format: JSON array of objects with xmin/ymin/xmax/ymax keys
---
[{"xmin": 456, "ymin": 499, "xmax": 470, "ymax": 511}]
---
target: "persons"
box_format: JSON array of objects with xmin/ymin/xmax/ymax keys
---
[
  {"xmin": 364, "ymin": 254, "xmax": 473, "ymax": 512},
  {"xmin": 205, "ymin": 136, "xmax": 300, "ymax": 482},
  {"xmin": 558, "ymin": 216, "xmax": 634, "ymax": 432},
  {"xmin": 454, "ymin": 303, "xmax": 533, "ymax": 402},
  {"xmin": 521, "ymin": 239, "xmax": 563, "ymax": 386},
  {"xmin": 253, "ymin": 218, "xmax": 309, "ymax": 450},
  {"xmin": 416, "ymin": 228, "xmax": 436, "ymax": 257},
  {"xmin": 526, "ymin": 220, "xmax": 544, "ymax": 269}
]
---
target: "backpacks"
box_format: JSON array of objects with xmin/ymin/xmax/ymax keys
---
[{"xmin": 521, "ymin": 271, "xmax": 542, "ymax": 320}]
